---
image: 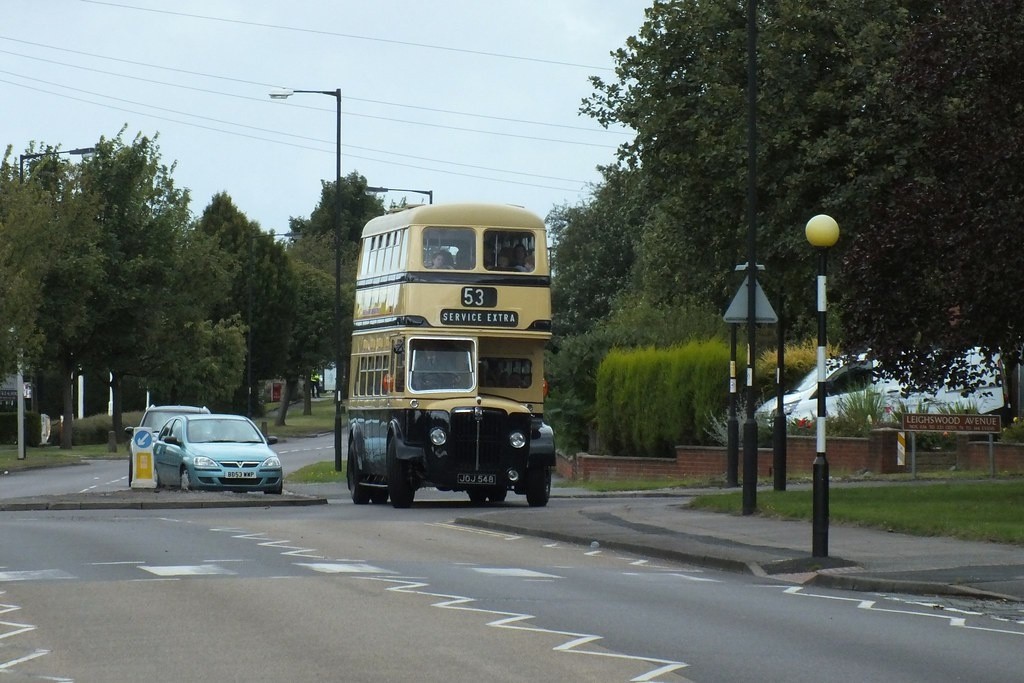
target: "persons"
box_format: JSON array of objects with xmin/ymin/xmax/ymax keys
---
[
  {"xmin": 502, "ymin": 247, "xmax": 517, "ymax": 267},
  {"xmin": 310, "ymin": 367, "xmax": 321, "ymax": 398},
  {"xmin": 188, "ymin": 424, "xmax": 204, "ymax": 442},
  {"xmin": 23, "ymin": 383, "xmax": 31, "ymax": 410},
  {"xmin": 482, "ymin": 240, "xmax": 494, "ymax": 268},
  {"xmin": 513, "ymin": 244, "xmax": 534, "ymax": 271},
  {"xmin": 478, "ymin": 358, "xmax": 508, "ymax": 386},
  {"xmin": 505, "ymin": 373, "xmax": 525, "ymax": 388},
  {"xmin": 426, "ymin": 253, "xmax": 456, "ymax": 269},
  {"xmin": 496, "ymin": 255, "xmax": 509, "ymax": 267},
  {"xmin": 455, "ymin": 248, "xmax": 472, "ymax": 269}
]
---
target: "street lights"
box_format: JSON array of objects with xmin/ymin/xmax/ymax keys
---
[
  {"xmin": 363, "ymin": 182, "xmax": 436, "ymax": 204},
  {"xmin": 268, "ymin": 85, "xmax": 344, "ymax": 471},
  {"xmin": 244, "ymin": 231, "xmax": 308, "ymax": 421},
  {"xmin": 16, "ymin": 146, "xmax": 101, "ymax": 461}
]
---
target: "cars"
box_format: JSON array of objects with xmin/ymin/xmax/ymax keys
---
[
  {"xmin": 129, "ymin": 404, "xmax": 212, "ymax": 485},
  {"xmin": 149, "ymin": 414, "xmax": 284, "ymax": 494}
]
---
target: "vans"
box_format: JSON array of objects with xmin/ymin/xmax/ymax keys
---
[{"xmin": 756, "ymin": 344, "xmax": 1004, "ymax": 429}]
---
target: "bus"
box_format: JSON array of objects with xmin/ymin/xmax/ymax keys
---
[{"xmin": 342, "ymin": 202, "xmax": 557, "ymax": 508}]
